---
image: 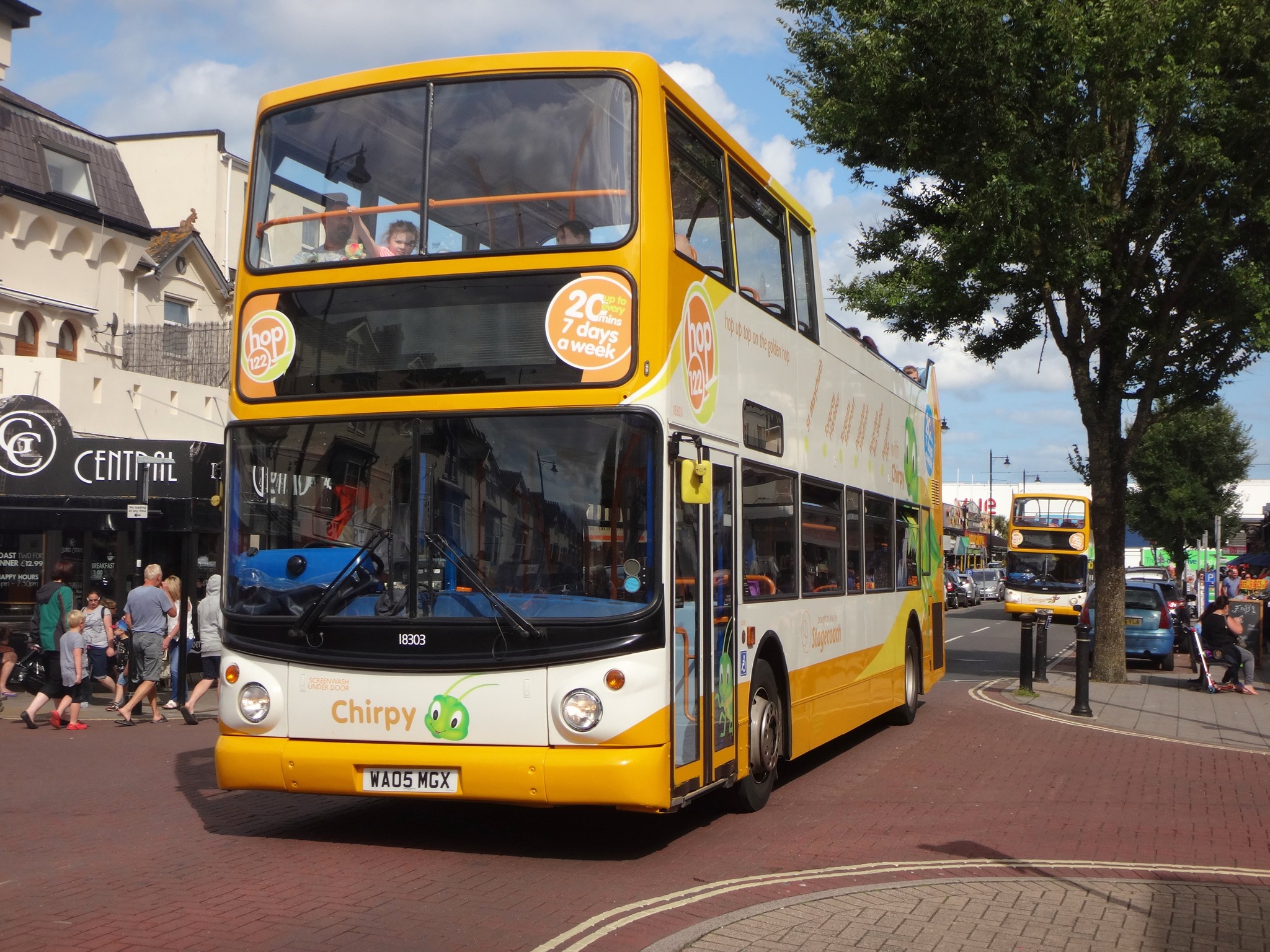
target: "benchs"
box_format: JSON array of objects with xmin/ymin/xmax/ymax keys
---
[{"xmin": 1182, "ymin": 627, "xmax": 1232, "ymax": 692}]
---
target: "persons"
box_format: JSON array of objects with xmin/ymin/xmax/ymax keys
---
[
  {"xmin": 676, "ymin": 231, "xmax": 694, "ymax": 259},
  {"xmin": 903, "ymin": 365, "xmax": 918, "ymax": 381},
  {"xmin": 178, "ymin": 573, "xmax": 225, "ymax": 724},
  {"xmin": 847, "ymin": 569, "xmax": 857, "ymax": 590},
  {"xmin": 1194, "ymin": 596, "xmax": 1259, "ymax": 695},
  {"xmin": 951, "ymin": 564, "xmax": 964, "ymax": 575},
  {"xmin": 347, "ymin": 205, "xmax": 417, "ymax": 257},
  {"xmin": 842, "ymin": 326, "xmax": 881, "ymax": 361},
  {"xmin": 293, "ymin": 200, "xmax": 367, "ymax": 265},
  {"xmin": 1185, "ymin": 564, "xmax": 1270, "ymax": 605},
  {"xmin": 557, "ymin": 221, "xmax": 592, "ymax": 245},
  {"xmin": 589, "ymin": 565, "xmax": 618, "ymax": 600},
  {"xmin": 20, "ymin": 561, "xmax": 194, "ymax": 730},
  {"xmin": 0, "ymin": 638, "xmax": 18, "ymax": 696},
  {"xmin": 1016, "ymin": 557, "xmax": 1033, "ymax": 573}
]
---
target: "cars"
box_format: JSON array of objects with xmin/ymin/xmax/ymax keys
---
[
  {"xmin": 957, "ymin": 573, "xmax": 981, "ymax": 607},
  {"xmin": 1072, "ymin": 581, "xmax": 1175, "ymax": 671},
  {"xmin": 972, "ymin": 568, "xmax": 1006, "ymax": 602},
  {"xmin": 988, "ymin": 562, "xmax": 1007, "ymax": 578},
  {"xmin": 943, "ymin": 579, "xmax": 948, "ymax": 611}
]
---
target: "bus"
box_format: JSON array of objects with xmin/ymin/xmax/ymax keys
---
[
  {"xmin": 210, "ymin": 49, "xmax": 947, "ymax": 815},
  {"xmin": 1004, "ymin": 494, "xmax": 1096, "ymax": 625}
]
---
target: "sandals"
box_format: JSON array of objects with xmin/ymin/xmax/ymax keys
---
[
  {"xmin": 162, "ymin": 700, "xmax": 177, "ymax": 709},
  {"xmin": 1242, "ymin": 687, "xmax": 1259, "ymax": 695},
  {"xmin": 50, "ymin": 710, "xmax": 61, "ymax": 728},
  {"xmin": 67, "ymin": 722, "xmax": 87, "ymax": 730}
]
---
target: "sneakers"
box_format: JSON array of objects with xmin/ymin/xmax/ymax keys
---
[{"xmin": 105, "ymin": 702, "xmax": 120, "ymax": 711}]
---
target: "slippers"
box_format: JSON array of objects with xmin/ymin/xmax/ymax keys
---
[
  {"xmin": 150, "ymin": 714, "xmax": 169, "ymax": 723},
  {"xmin": 114, "ymin": 718, "xmax": 136, "ymax": 726},
  {"xmin": 180, "ymin": 705, "xmax": 199, "ymax": 725},
  {"xmin": 2, "ymin": 690, "xmax": 17, "ymax": 697}
]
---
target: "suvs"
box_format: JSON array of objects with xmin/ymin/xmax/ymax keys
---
[
  {"xmin": 1126, "ymin": 578, "xmax": 1197, "ymax": 654},
  {"xmin": 1124, "ymin": 566, "xmax": 1171, "ymax": 583},
  {"xmin": 943, "ymin": 569, "xmax": 968, "ymax": 608}
]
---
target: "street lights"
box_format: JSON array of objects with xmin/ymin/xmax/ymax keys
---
[
  {"xmin": 989, "ymin": 448, "xmax": 1012, "ymax": 563},
  {"xmin": 1023, "ymin": 469, "xmax": 1041, "ymax": 516}
]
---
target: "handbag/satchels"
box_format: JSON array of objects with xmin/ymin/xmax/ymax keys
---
[{"xmin": 5, "ymin": 650, "xmax": 48, "ymax": 696}]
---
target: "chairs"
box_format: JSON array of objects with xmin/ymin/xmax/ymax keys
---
[
  {"xmin": 700, "ymin": 266, "xmax": 809, "ymax": 333},
  {"xmin": 1125, "ymin": 591, "xmax": 1136, "ymax": 602},
  {"xmin": 676, "ymin": 575, "xmax": 839, "ymax": 608},
  {"xmin": 1138, "ymin": 592, "xmax": 1151, "ymax": 603},
  {"xmin": 1014, "ymin": 518, "xmax": 1084, "ymax": 528}
]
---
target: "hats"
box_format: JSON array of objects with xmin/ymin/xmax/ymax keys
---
[{"xmin": 109, "ymin": 620, "xmax": 129, "ymax": 631}]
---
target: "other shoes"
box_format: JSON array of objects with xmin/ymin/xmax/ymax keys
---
[
  {"xmin": 21, "ymin": 711, "xmax": 38, "ymax": 728},
  {"xmin": 60, "ymin": 719, "xmax": 70, "ymax": 726}
]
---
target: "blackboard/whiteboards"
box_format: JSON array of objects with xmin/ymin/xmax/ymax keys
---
[{"xmin": 1227, "ymin": 598, "xmax": 1263, "ymax": 668}]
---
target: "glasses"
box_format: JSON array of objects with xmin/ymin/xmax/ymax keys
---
[
  {"xmin": 87, "ymin": 598, "xmax": 100, "ymax": 601},
  {"xmin": 1245, "ymin": 577, "xmax": 1251, "ymax": 578},
  {"xmin": 1231, "ymin": 569, "xmax": 1238, "ymax": 574}
]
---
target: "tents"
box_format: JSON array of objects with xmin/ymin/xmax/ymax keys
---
[{"xmin": 1224, "ymin": 553, "xmax": 1270, "ymax": 566}]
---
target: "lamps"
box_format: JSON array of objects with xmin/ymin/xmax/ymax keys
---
[
  {"xmin": 330, "ymin": 141, "xmax": 371, "ymax": 190},
  {"xmin": 92, "ymin": 313, "xmax": 117, "ymax": 337}
]
